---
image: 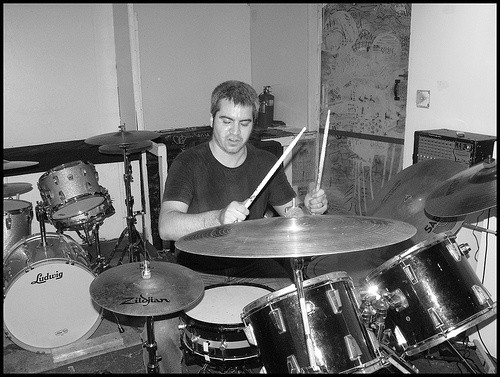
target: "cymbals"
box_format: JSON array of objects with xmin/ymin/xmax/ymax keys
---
[
  {"xmin": 367, "ymin": 158, "xmax": 471, "ymax": 243},
  {"xmin": 3, "ymin": 182, "xmax": 33, "ymax": 198},
  {"xmin": 84, "ymin": 131, "xmax": 162, "ymax": 145},
  {"xmin": 89, "ymin": 261, "xmax": 204, "ymax": 317},
  {"xmin": 99, "ymin": 141, "xmax": 153, "ymax": 154},
  {"xmin": 425, "ymin": 160, "xmax": 497, "ymax": 218},
  {"xmin": 174, "ymin": 215, "xmax": 418, "ymax": 258},
  {"xmin": 4, "ymin": 159, "xmax": 39, "ymax": 171}
]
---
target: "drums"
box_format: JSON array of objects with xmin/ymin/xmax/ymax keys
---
[
  {"xmin": 53, "ymin": 185, "xmax": 116, "ymax": 231},
  {"xmin": 179, "ymin": 281, "xmax": 276, "ymax": 366},
  {"xmin": 3, "ymin": 233, "xmax": 105, "ymax": 354},
  {"xmin": 360, "ymin": 231, "xmax": 496, "ymax": 359},
  {"xmin": 240, "ymin": 272, "xmax": 388, "ymax": 374},
  {"xmin": 38, "ymin": 160, "xmax": 105, "ymax": 221},
  {"xmin": 304, "ymin": 239, "xmax": 415, "ymax": 286},
  {"xmin": 3, "ymin": 199, "xmax": 34, "ymax": 258}
]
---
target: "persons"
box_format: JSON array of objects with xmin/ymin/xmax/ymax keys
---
[{"xmin": 152, "ymin": 81, "xmax": 328, "ymax": 374}]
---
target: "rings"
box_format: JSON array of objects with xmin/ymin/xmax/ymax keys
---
[
  {"xmin": 321, "ymin": 202, "xmax": 324, "ymax": 207},
  {"xmin": 233, "ymin": 219, "xmax": 238, "ymax": 222}
]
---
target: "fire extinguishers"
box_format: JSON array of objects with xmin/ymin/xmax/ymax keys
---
[{"xmin": 258, "ymin": 85, "xmax": 274, "ymax": 128}]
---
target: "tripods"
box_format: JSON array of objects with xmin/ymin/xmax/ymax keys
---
[{"xmin": 102, "ymin": 145, "xmax": 153, "ymax": 275}]
